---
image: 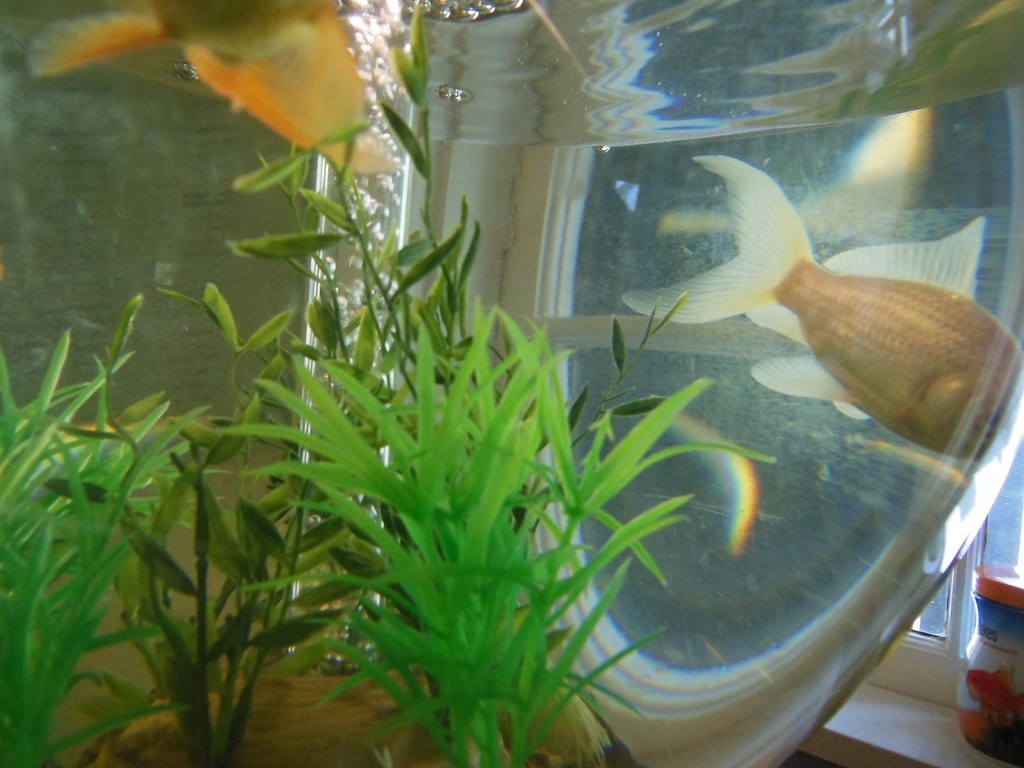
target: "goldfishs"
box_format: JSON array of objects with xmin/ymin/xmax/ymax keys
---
[
  {"xmin": 29, "ymin": 0, "xmax": 402, "ymax": 181},
  {"xmin": 615, "ymin": 153, "xmax": 1019, "ymax": 462}
]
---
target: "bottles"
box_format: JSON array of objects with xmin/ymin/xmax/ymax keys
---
[{"xmin": 957, "ymin": 564, "xmax": 1024, "ymax": 763}]
